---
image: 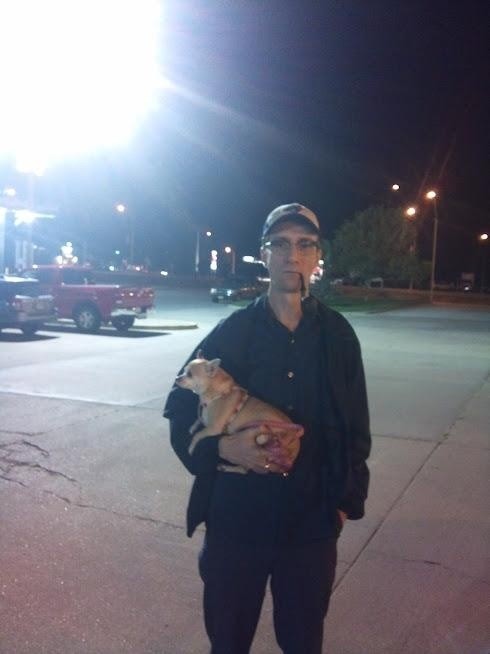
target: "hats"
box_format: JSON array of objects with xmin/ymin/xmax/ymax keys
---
[{"xmin": 262, "ymin": 203, "xmax": 321, "ymax": 237}]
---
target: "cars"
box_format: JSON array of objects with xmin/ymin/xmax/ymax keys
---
[
  {"xmin": 210, "ymin": 280, "xmax": 258, "ymax": 304},
  {"xmin": 1, "ymin": 273, "xmax": 59, "ymax": 337}
]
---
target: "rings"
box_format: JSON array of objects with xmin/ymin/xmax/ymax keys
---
[{"xmin": 264, "ymin": 463, "xmax": 271, "ymax": 470}]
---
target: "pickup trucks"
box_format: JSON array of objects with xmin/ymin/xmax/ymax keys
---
[{"xmin": 22, "ymin": 264, "xmax": 156, "ymax": 332}]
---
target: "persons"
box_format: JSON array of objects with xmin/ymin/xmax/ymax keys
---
[{"xmin": 160, "ymin": 202, "xmax": 372, "ymax": 653}]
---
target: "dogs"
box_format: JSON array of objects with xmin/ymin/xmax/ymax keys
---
[{"xmin": 174, "ymin": 349, "xmax": 304, "ymax": 475}]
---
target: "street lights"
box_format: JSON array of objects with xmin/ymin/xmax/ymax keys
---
[
  {"xmin": 196, "ymin": 229, "xmax": 211, "ymax": 272},
  {"xmin": 427, "ymin": 191, "xmax": 439, "ymax": 289},
  {"xmin": 225, "ymin": 248, "xmax": 235, "ymax": 276},
  {"xmin": 117, "ymin": 204, "xmax": 134, "ymax": 263},
  {"xmin": 407, "ymin": 207, "xmax": 418, "ymax": 289}
]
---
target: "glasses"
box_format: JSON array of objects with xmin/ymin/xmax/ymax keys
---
[{"xmin": 262, "ymin": 239, "xmax": 318, "ymax": 256}]
